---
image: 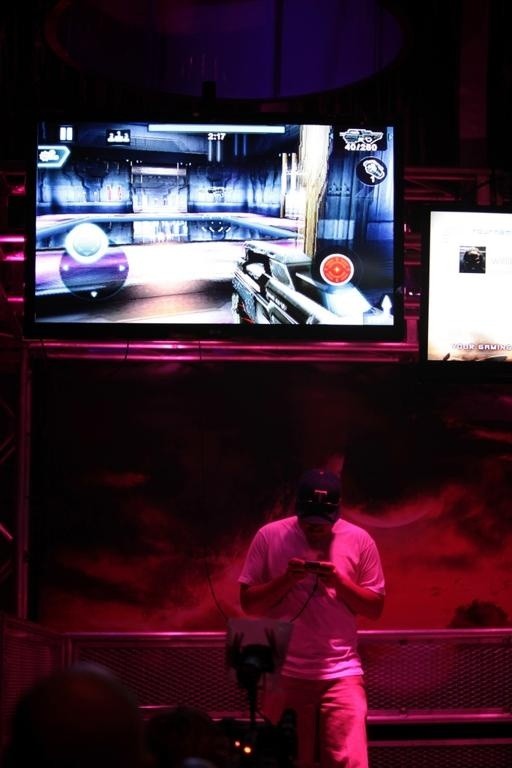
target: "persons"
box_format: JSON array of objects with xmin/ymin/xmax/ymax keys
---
[
  {"xmin": 141, "ymin": 705, "xmax": 231, "ymax": 767},
  {"xmin": 6, "ymin": 660, "xmax": 143, "ymax": 768},
  {"xmin": 236, "ymin": 461, "xmax": 389, "ymax": 768}
]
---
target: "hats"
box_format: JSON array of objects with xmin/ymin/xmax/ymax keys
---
[{"xmin": 295, "ymin": 469, "xmax": 342, "ymax": 527}]
